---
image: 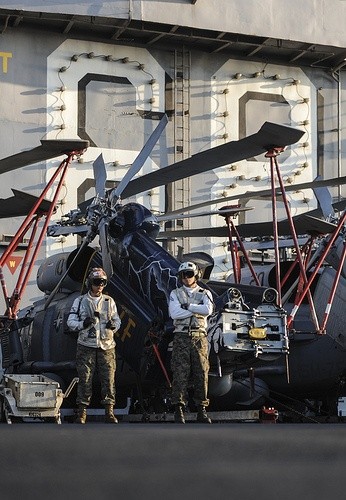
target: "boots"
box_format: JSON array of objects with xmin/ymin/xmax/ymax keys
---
[
  {"xmin": 174, "ymin": 406, "xmax": 185, "ymax": 424},
  {"xmin": 105, "ymin": 404, "xmax": 118, "ymax": 424},
  {"xmin": 76, "ymin": 404, "xmax": 86, "ymax": 424},
  {"xmin": 197, "ymin": 407, "xmax": 211, "ymax": 423}
]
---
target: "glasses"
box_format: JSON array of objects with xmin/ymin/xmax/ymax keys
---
[
  {"xmin": 92, "ymin": 279, "xmax": 107, "ymax": 286},
  {"xmin": 179, "ymin": 270, "xmax": 195, "ymax": 278}
]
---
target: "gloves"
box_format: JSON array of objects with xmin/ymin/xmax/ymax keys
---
[
  {"xmin": 106, "ymin": 320, "xmax": 116, "ymax": 330},
  {"xmin": 181, "ymin": 304, "xmax": 188, "ymax": 310},
  {"xmin": 83, "ymin": 318, "xmax": 96, "ymax": 328}
]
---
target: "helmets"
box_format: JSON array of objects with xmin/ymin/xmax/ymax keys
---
[
  {"xmin": 88, "ymin": 267, "xmax": 107, "ymax": 283},
  {"xmin": 178, "ymin": 262, "xmax": 198, "ymax": 276}
]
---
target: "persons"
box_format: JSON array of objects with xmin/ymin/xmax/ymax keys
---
[
  {"xmin": 67, "ymin": 268, "xmax": 119, "ymax": 424},
  {"xmin": 168, "ymin": 261, "xmax": 213, "ymax": 424}
]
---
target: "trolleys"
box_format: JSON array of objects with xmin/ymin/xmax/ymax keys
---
[{"xmin": 0, "ymin": 374, "xmax": 79, "ymax": 424}]
